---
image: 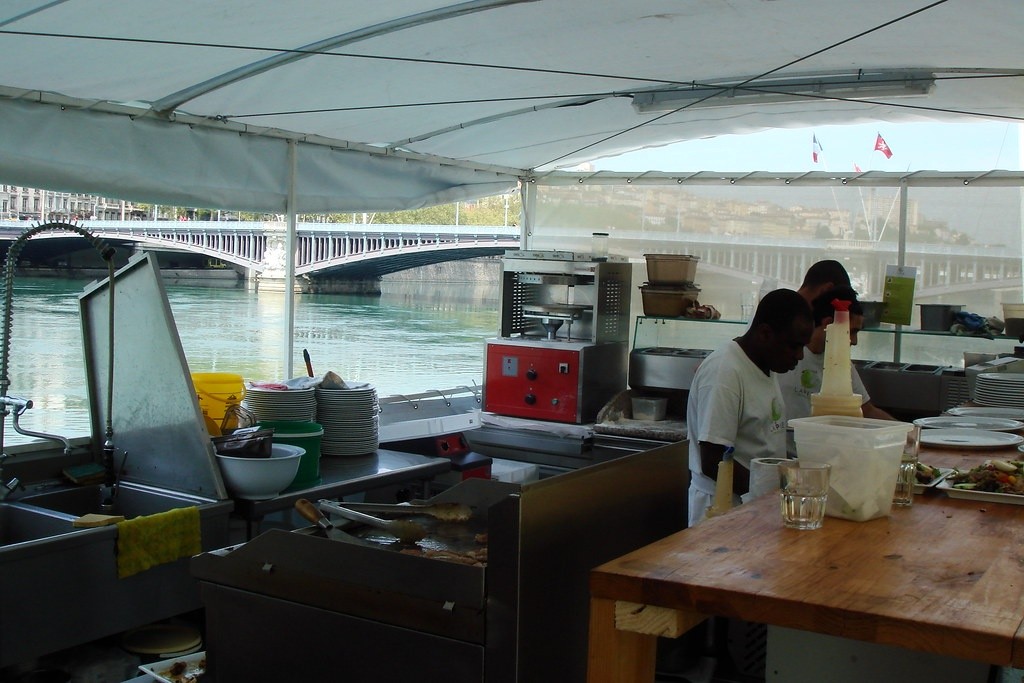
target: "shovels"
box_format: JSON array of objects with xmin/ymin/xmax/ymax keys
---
[{"xmin": 293, "ymin": 498, "xmax": 369, "ymax": 546}]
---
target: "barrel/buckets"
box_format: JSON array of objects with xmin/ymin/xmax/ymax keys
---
[
  {"xmin": 121, "ymin": 624, "xmax": 202, "ymax": 665},
  {"xmin": 249, "ymin": 422, "xmax": 324, "ymax": 485},
  {"xmin": 190, "ymin": 372, "xmax": 247, "ymax": 438}
]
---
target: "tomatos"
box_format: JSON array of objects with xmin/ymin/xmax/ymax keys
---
[{"xmin": 997, "ymin": 473, "xmax": 1010, "ymax": 483}]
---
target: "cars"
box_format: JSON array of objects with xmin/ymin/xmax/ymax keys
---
[
  {"xmin": 56, "ymin": 259, "xmax": 68, "ymax": 268},
  {"xmin": 208, "ymin": 258, "xmax": 227, "ymax": 269},
  {"xmin": 132, "ymin": 217, "xmax": 143, "ymax": 222},
  {"xmin": 20, "ymin": 258, "xmax": 32, "ymax": 267}
]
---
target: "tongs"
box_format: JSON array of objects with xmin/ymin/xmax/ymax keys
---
[{"xmin": 317, "ymin": 499, "xmax": 475, "ymax": 542}]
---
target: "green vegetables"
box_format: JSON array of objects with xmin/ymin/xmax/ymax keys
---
[
  {"xmin": 945, "ymin": 461, "xmax": 1024, "ymax": 493},
  {"xmin": 916, "ymin": 462, "xmax": 941, "ymax": 477}
]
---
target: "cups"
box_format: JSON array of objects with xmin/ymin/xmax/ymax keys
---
[
  {"xmin": 891, "ymin": 425, "xmax": 921, "ymax": 506},
  {"xmin": 778, "ymin": 461, "xmax": 833, "ymax": 530},
  {"xmin": 1000, "ymin": 303, "xmax": 1024, "ymax": 337}
]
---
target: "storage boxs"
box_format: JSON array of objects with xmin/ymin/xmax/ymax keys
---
[
  {"xmin": 915, "ymin": 303, "xmax": 967, "ymax": 331},
  {"xmin": 638, "ymin": 254, "xmax": 700, "ymax": 314},
  {"xmin": 786, "ymin": 416, "xmax": 913, "ymax": 522}
]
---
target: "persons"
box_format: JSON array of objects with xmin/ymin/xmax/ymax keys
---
[
  {"xmin": 177, "ymin": 215, "xmax": 187, "ymax": 221},
  {"xmin": 776, "ymin": 260, "xmax": 897, "ymax": 458},
  {"xmin": 687, "ymin": 289, "xmax": 814, "ymax": 683}
]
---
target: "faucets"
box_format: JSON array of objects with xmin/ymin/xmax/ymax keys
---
[{"xmin": 4, "ymin": 395, "xmax": 74, "ymax": 455}]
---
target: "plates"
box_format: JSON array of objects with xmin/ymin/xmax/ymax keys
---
[
  {"xmin": 935, "ymin": 469, "xmax": 1024, "ymax": 505},
  {"xmin": 240, "ymin": 379, "xmax": 380, "ymax": 456},
  {"xmin": 947, "ymin": 407, "xmax": 1024, "ymax": 421},
  {"xmin": 895, "ymin": 464, "xmax": 955, "ymax": 495},
  {"xmin": 919, "ymin": 429, "xmax": 1024, "ymax": 449},
  {"xmin": 913, "ymin": 416, "xmax": 1024, "ymax": 431},
  {"xmin": 138, "ymin": 651, "xmax": 206, "ymax": 683},
  {"xmin": 1018, "ymin": 444, "xmax": 1024, "ymax": 453},
  {"xmin": 972, "ymin": 372, "xmax": 1024, "ymax": 409}
]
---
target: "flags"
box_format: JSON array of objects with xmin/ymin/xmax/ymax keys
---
[
  {"xmin": 813, "ymin": 135, "xmax": 822, "ymax": 163},
  {"xmin": 875, "ymin": 134, "xmax": 892, "ymax": 159}
]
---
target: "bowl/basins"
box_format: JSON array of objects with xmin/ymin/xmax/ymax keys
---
[{"xmin": 215, "ymin": 443, "xmax": 306, "ymax": 501}]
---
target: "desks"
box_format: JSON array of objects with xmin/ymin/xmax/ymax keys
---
[{"xmin": 585, "ymin": 405, "xmax": 1024, "ymax": 683}]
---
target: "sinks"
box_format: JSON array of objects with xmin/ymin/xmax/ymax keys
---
[
  {"xmin": 17, "ymin": 480, "xmax": 205, "ymax": 524},
  {"xmin": 0, "ymin": 502, "xmax": 92, "ymax": 550}
]
